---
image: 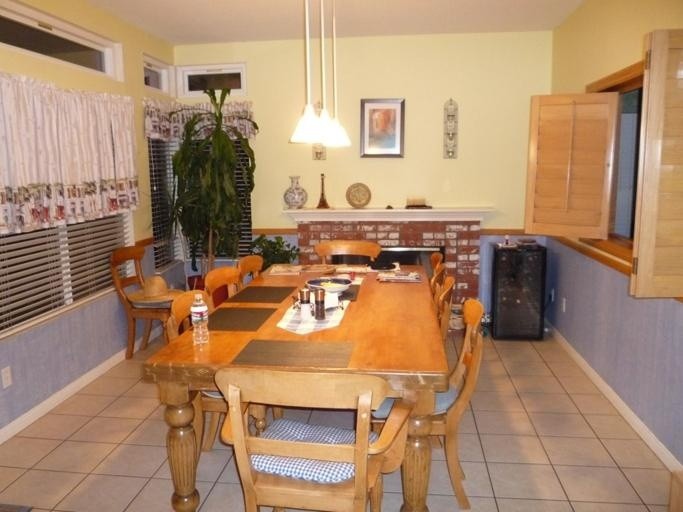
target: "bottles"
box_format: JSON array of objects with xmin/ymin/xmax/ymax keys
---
[
  {"xmin": 190, "ymin": 293, "xmax": 210, "ymax": 343},
  {"xmin": 299, "ymin": 288, "xmax": 311, "ymax": 320},
  {"xmin": 314, "ymin": 290, "xmax": 327, "ymax": 321}
]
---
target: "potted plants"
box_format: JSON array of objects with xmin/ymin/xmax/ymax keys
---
[{"xmin": 156, "ymin": 87, "xmax": 259, "ymax": 289}]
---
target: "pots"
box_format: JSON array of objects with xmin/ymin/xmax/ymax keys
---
[{"xmin": 304, "ymin": 277, "xmax": 352, "ymax": 296}]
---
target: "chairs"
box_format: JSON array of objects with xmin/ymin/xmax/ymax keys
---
[
  {"xmin": 110, "ymin": 246, "xmax": 191, "ymax": 360},
  {"xmin": 204, "ymin": 268, "xmax": 241, "ymax": 312},
  {"xmin": 428, "ymin": 249, "xmax": 456, "ymax": 352},
  {"xmin": 312, "ymin": 237, "xmax": 382, "ymax": 263},
  {"xmin": 165, "ymin": 288, "xmax": 284, "ymax": 468},
  {"xmin": 209, "ymin": 362, "xmax": 413, "ymax": 511},
  {"xmin": 239, "ymin": 255, "xmax": 264, "ymax": 288},
  {"xmin": 371, "ymin": 298, "xmax": 488, "ymax": 510}
]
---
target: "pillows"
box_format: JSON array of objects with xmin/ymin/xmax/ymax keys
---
[{"xmin": 247, "ymin": 417, "xmax": 375, "ymax": 486}]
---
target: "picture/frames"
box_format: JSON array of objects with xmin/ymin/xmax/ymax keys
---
[{"xmin": 358, "ymin": 99, "xmax": 406, "ymax": 157}]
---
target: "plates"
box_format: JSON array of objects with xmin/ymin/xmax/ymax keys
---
[{"xmin": 346, "ymin": 183, "xmax": 370, "ymax": 209}]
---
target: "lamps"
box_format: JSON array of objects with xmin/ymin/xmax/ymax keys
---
[{"xmin": 288, "ymin": 0, "xmax": 352, "ymax": 150}]
